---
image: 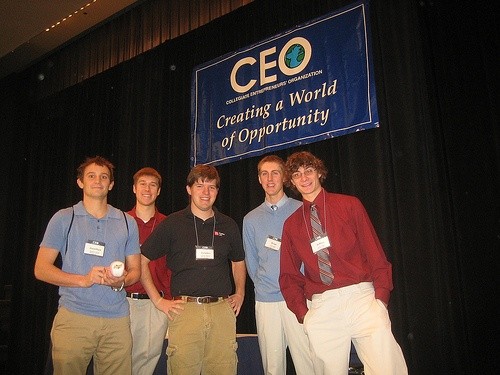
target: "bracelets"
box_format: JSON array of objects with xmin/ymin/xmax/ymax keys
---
[
  {"xmin": 111, "ymin": 281, "xmax": 125, "ymax": 292},
  {"xmin": 155, "ymin": 297, "xmax": 163, "ymax": 306}
]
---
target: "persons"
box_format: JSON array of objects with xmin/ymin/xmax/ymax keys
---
[
  {"xmin": 127, "ymin": 168, "xmax": 171, "ymax": 375},
  {"xmin": 279, "ymin": 152, "xmax": 408, "ymax": 375},
  {"xmin": 242, "ymin": 156, "xmax": 310, "ymax": 375},
  {"xmin": 141, "ymin": 166, "xmax": 246, "ymax": 375},
  {"xmin": 35, "ymin": 159, "xmax": 140, "ymax": 375}
]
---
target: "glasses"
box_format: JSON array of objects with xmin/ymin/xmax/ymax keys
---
[{"xmin": 291, "ymin": 167, "xmax": 317, "ymax": 178}]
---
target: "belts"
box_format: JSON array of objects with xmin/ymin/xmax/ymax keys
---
[
  {"xmin": 174, "ymin": 295, "xmax": 228, "ymax": 303},
  {"xmin": 126, "ymin": 291, "xmax": 163, "ymax": 299}
]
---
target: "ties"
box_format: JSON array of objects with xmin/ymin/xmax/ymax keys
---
[
  {"xmin": 310, "ymin": 202, "xmax": 334, "ymax": 286},
  {"xmin": 271, "ymin": 204, "xmax": 278, "ymax": 211}
]
